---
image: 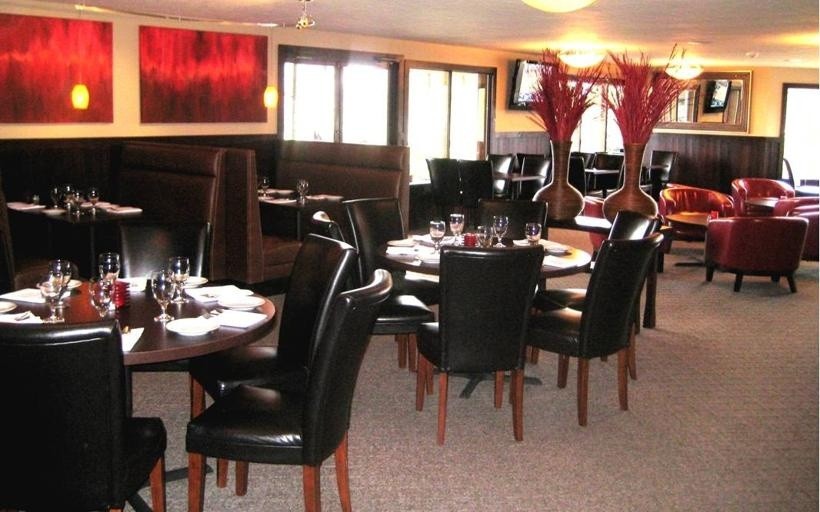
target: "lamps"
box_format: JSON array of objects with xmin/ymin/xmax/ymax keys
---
[
  {"xmin": 259, "ymin": 19, "xmax": 283, "ymax": 111},
  {"xmin": 68, "ymin": 5, "xmax": 92, "ymax": 116},
  {"xmin": 289, "ymin": 0, "xmax": 320, "ymax": 34}
]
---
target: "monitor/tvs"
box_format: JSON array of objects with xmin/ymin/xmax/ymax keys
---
[
  {"xmin": 704, "ymin": 81, "xmax": 732, "ymax": 113},
  {"xmin": 508, "ymin": 58, "xmax": 559, "ymax": 111}
]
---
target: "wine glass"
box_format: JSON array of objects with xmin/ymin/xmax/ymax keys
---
[
  {"xmin": 429, "ymin": 218, "xmax": 447, "ymax": 256},
  {"xmin": 260, "ymin": 176, "xmax": 273, "ymax": 199},
  {"xmin": 149, "ymin": 270, "xmax": 177, "ymax": 324},
  {"xmin": 49, "ymin": 185, "xmax": 102, "ymax": 216},
  {"xmin": 448, "ymin": 213, "xmax": 468, "ymax": 244},
  {"xmin": 491, "ymin": 214, "xmax": 509, "ymax": 248},
  {"xmin": 165, "ymin": 254, "xmax": 193, "ymax": 303},
  {"xmin": 36, "ymin": 273, "xmax": 68, "ymax": 326},
  {"xmin": 297, "ymin": 177, "xmax": 309, "ymax": 203}
]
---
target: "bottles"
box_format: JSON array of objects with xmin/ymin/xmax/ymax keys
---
[{"xmin": 707, "ymin": 211, "xmax": 720, "ymax": 224}]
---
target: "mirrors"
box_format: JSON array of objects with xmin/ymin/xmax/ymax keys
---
[{"xmin": 632, "ymin": 68, "xmax": 752, "ymax": 137}]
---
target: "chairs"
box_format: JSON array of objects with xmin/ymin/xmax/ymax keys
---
[
  {"xmin": 3, "ymin": 318, "xmax": 168, "ymax": 511},
  {"xmin": 313, "ymin": 210, "xmax": 436, "ymax": 398},
  {"xmin": 583, "ymin": 177, "xmax": 818, "ymax": 296},
  {"xmin": 343, "ymin": 196, "xmax": 444, "ymax": 367},
  {"xmin": 424, "ymin": 151, "xmax": 677, "ymax": 230},
  {"xmin": 530, "ymin": 208, "xmax": 654, "ymax": 382},
  {"xmin": 185, "ymin": 268, "xmax": 395, "ymax": 507},
  {"xmin": 3, "ymin": 194, "xmax": 80, "ymax": 291},
  {"xmin": 415, "ymin": 243, "xmax": 545, "ymax": 448},
  {"xmin": 189, "ymin": 230, "xmax": 357, "ymax": 487},
  {"xmin": 214, "ymin": 147, "xmax": 303, "ymax": 283},
  {"xmin": 509, "ymin": 233, "xmax": 654, "ymax": 428},
  {"xmin": 107, "ymin": 139, "xmax": 226, "ymax": 286},
  {"xmin": 273, "ymin": 141, "xmax": 410, "ymax": 242}
]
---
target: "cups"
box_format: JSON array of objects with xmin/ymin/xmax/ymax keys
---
[
  {"xmin": 51, "ymin": 260, "xmax": 74, "ymax": 285},
  {"xmin": 525, "ymin": 221, "xmax": 541, "ymax": 246},
  {"xmin": 88, "ymin": 278, "xmax": 114, "ymax": 319},
  {"xmin": 95, "ymin": 251, "xmax": 121, "ymax": 281},
  {"xmin": 476, "ymin": 222, "xmax": 492, "ymax": 246}
]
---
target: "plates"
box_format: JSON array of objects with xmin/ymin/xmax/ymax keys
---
[
  {"xmin": 0, "ymin": 301, "xmax": 17, "ymax": 313},
  {"xmin": 35, "ymin": 280, "xmax": 82, "ymax": 293},
  {"xmin": 41, "ymin": 208, "xmax": 67, "ymax": 217},
  {"xmin": 388, "ymin": 238, "xmax": 416, "ymax": 249},
  {"xmin": 119, "ymin": 274, "xmax": 152, "ymax": 294},
  {"xmin": 163, "ymin": 316, "xmax": 217, "ymax": 339},
  {"xmin": 414, "ymin": 253, "xmax": 440, "ymax": 265},
  {"xmin": 167, "ymin": 275, "xmax": 210, "ymax": 289},
  {"xmin": 218, "ymin": 295, "xmax": 268, "ymax": 314}
]
